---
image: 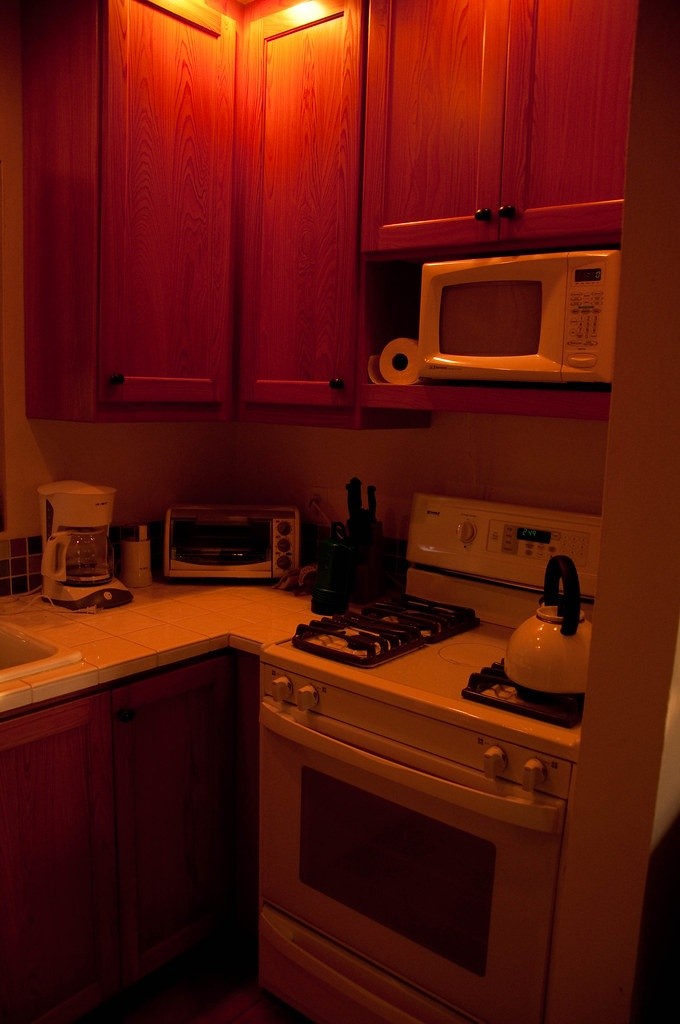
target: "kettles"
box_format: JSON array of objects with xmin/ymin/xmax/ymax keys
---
[
  {"xmin": 503, "ymin": 552, "xmax": 592, "ymax": 701},
  {"xmin": 35, "ymin": 473, "xmax": 135, "ymax": 611}
]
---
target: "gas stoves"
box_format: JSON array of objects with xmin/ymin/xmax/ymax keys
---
[{"xmin": 260, "ymin": 487, "xmax": 599, "ymax": 804}]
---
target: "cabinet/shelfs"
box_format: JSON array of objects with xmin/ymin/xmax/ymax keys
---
[
  {"xmin": 20, "ymin": 0, "xmax": 639, "ymax": 433},
  {"xmin": 2, "ymin": 563, "xmax": 602, "ymax": 1024}
]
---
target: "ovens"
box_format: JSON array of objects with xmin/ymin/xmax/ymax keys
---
[{"xmin": 250, "ymin": 697, "xmax": 566, "ymax": 1024}]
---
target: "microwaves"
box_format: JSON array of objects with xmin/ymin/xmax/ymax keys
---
[
  {"xmin": 415, "ymin": 250, "xmax": 618, "ymax": 395},
  {"xmin": 163, "ymin": 498, "xmax": 300, "ymax": 587}
]
